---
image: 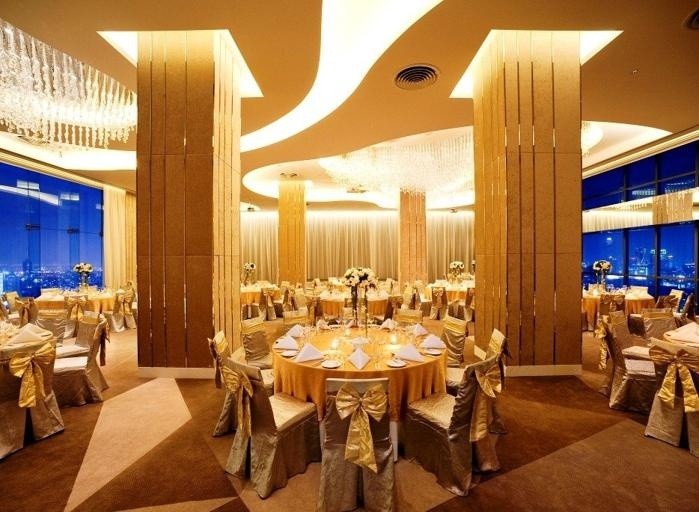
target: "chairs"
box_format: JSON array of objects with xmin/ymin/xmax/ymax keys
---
[
  {"xmin": 3, "ymin": 339, "xmax": 65, "ymax": 456},
  {"xmin": 445, "ymin": 327, "xmax": 507, "ymax": 392},
  {"xmin": 405, "ymin": 353, "xmax": 502, "ymax": 498},
  {"xmin": 5, "ymin": 279, "xmax": 138, "ymax": 332},
  {"xmin": 206, "ymin": 332, "xmax": 275, "ymax": 435},
  {"xmin": 580, "ymin": 272, "xmax": 698, "ymax": 455},
  {"xmin": 237, "ymin": 269, "xmax": 477, "ymax": 330},
  {"xmin": 54, "ymin": 315, "xmax": 110, "ymax": 406},
  {"xmin": 442, "ymin": 314, "xmax": 469, "ymax": 363},
  {"xmin": 242, "ymin": 331, "xmax": 277, "ymax": 371},
  {"xmin": 222, "ymin": 356, "xmax": 318, "ymax": 501},
  {"xmin": 318, "ymin": 378, "xmax": 398, "ymax": 511}
]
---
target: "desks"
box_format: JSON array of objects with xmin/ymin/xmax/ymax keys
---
[
  {"xmin": 0, "ymin": 321, "xmax": 55, "ymax": 357},
  {"xmin": 271, "ymin": 322, "xmax": 445, "ymax": 406}
]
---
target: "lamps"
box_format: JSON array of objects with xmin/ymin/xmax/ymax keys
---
[
  {"xmin": 2, "ymin": 22, "xmax": 140, "ymax": 153},
  {"xmin": 316, "ymin": 104, "xmax": 606, "ymax": 201}
]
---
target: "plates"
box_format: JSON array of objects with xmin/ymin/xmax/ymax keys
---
[{"xmin": 281, "ymin": 348, "xmax": 442, "ymax": 369}]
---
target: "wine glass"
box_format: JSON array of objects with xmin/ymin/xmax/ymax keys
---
[{"xmin": 313, "ymin": 312, "xmax": 375, "ymax": 336}]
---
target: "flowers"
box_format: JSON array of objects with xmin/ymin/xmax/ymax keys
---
[
  {"xmin": 243, "ymin": 262, "xmax": 256, "ymax": 271},
  {"xmin": 593, "ymin": 259, "xmax": 612, "ymax": 271},
  {"xmin": 73, "ymin": 262, "xmax": 92, "ymax": 272},
  {"xmin": 449, "ymin": 261, "xmax": 464, "ymax": 271},
  {"xmin": 343, "ymin": 265, "xmax": 376, "ymax": 286}
]
